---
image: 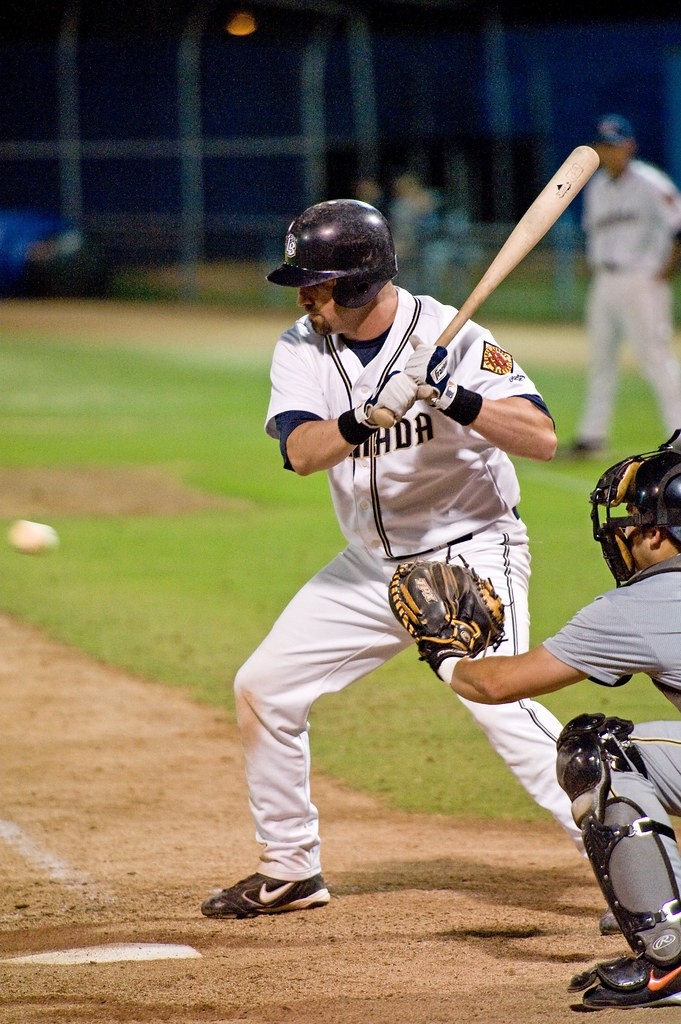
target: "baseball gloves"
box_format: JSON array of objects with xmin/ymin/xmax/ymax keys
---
[{"xmin": 388, "ymin": 556, "xmax": 507, "ymax": 663}]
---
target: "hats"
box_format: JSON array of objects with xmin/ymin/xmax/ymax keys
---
[{"xmin": 590, "ymin": 114, "xmax": 632, "ymax": 148}]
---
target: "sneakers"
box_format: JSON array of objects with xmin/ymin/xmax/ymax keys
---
[
  {"xmin": 201, "ymin": 871, "xmax": 331, "ymax": 918},
  {"xmin": 582, "ymin": 961, "xmax": 681, "ymax": 1010},
  {"xmin": 599, "ymin": 904, "xmax": 623, "ymax": 935}
]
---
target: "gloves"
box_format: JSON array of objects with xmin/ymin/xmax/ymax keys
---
[
  {"xmin": 401, "ymin": 335, "xmax": 483, "ymax": 426},
  {"xmin": 337, "ymin": 369, "xmax": 431, "ymax": 446}
]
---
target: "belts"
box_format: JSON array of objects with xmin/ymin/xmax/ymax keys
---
[{"xmin": 394, "ymin": 506, "xmax": 520, "ymax": 561}]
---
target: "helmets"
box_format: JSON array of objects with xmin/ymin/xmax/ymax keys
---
[
  {"xmin": 588, "ymin": 446, "xmax": 681, "ymax": 542},
  {"xmin": 264, "ymin": 199, "xmax": 397, "ymax": 309}
]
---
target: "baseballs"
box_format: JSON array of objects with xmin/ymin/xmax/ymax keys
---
[{"xmin": 10, "ymin": 523, "xmax": 44, "ymax": 553}]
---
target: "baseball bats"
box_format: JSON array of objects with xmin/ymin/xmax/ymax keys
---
[{"xmin": 372, "ymin": 143, "xmax": 605, "ymax": 431}]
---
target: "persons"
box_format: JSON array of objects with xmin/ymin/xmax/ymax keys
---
[
  {"xmin": 562, "ymin": 117, "xmax": 681, "ymax": 460},
  {"xmin": 389, "ymin": 429, "xmax": 681, "ymax": 1012},
  {"xmin": 355, "ymin": 173, "xmax": 455, "ymax": 296},
  {"xmin": 200, "ymin": 198, "xmax": 628, "ymax": 933}
]
